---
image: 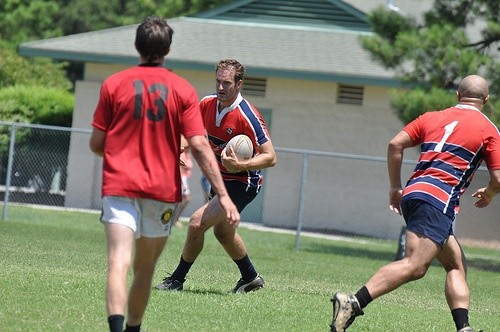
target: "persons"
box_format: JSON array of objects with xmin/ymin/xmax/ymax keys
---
[
  {"xmin": 173, "ymin": 133, "xmax": 194, "ymax": 228},
  {"xmin": 328, "ymin": 74, "xmax": 500, "ymax": 332},
  {"xmin": 153, "ymin": 58, "xmax": 278, "ymax": 293},
  {"xmin": 89, "ymin": 15, "xmax": 240, "ymax": 332},
  {"xmin": 200, "ymin": 174, "xmax": 212, "ymax": 205}
]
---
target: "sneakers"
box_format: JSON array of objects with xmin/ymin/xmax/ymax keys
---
[
  {"xmin": 457, "ymin": 326, "xmax": 475, "ymax": 332},
  {"xmin": 155, "ymin": 273, "xmax": 186, "ymax": 291},
  {"xmin": 233, "ymin": 273, "xmax": 265, "ymax": 294},
  {"xmin": 329, "ymin": 291, "xmax": 364, "ymax": 332}
]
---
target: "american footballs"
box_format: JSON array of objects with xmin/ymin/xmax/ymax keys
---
[{"xmin": 225, "ymin": 135, "xmax": 254, "ymax": 175}]
